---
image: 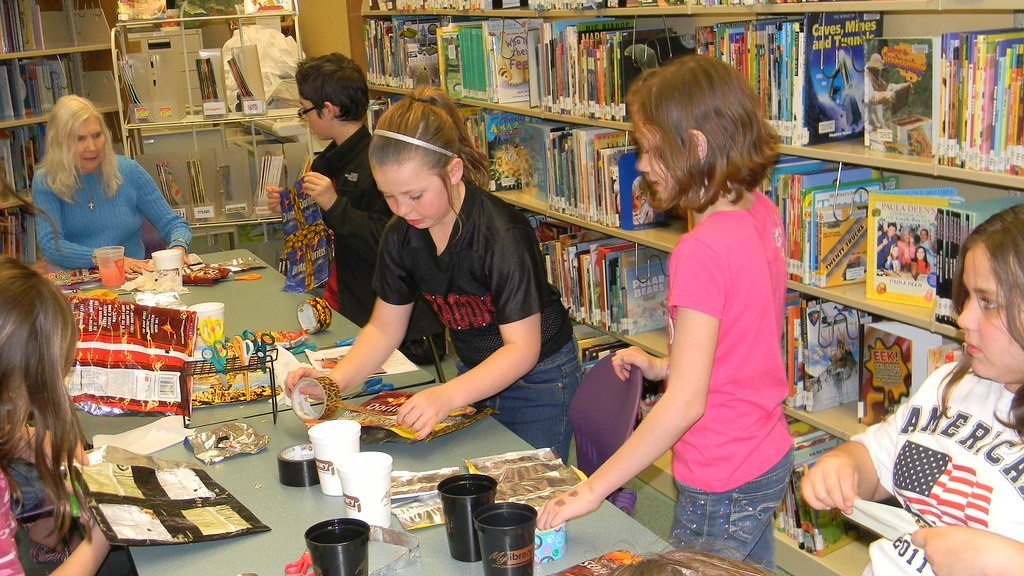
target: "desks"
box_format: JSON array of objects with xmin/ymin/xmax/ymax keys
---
[{"xmin": 34, "ymin": 250, "xmax": 677, "ymax": 576}]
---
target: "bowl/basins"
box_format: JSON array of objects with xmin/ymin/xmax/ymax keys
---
[{"xmin": 533, "ymin": 507, "xmax": 566, "ymax": 563}]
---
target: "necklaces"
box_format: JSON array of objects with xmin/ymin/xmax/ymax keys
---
[{"xmin": 89, "ymin": 201, "xmax": 96, "ymax": 209}]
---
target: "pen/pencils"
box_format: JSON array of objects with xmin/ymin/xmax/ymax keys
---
[{"xmin": 118, "ymin": 292, "xmax": 136, "ymax": 296}]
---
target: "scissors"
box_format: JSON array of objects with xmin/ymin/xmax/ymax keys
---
[
  {"xmin": 235, "ymin": 338, "xmax": 254, "ymax": 401},
  {"xmin": 202, "ymin": 340, "xmax": 232, "ymax": 390},
  {"xmin": 234, "ymin": 329, "xmax": 275, "ymax": 373},
  {"xmin": 225, "ymin": 338, "xmax": 239, "ymax": 357},
  {"xmin": 285, "ymin": 546, "xmax": 317, "ymax": 576},
  {"xmin": 218, "ymin": 273, "xmax": 262, "ymax": 282}
]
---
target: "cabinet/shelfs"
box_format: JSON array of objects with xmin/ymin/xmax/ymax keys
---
[
  {"xmin": 0, "ymin": 41, "xmax": 119, "ymax": 283},
  {"xmin": 113, "ymin": 15, "xmax": 314, "ymax": 230},
  {"xmin": 364, "ymin": 0, "xmax": 1024, "ymax": 576}
]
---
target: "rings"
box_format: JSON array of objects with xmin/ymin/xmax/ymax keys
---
[{"xmin": 131, "ymin": 265, "xmax": 136, "ymax": 270}]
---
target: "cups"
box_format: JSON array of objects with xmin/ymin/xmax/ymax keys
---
[
  {"xmin": 307, "ymin": 420, "xmax": 361, "ymax": 496},
  {"xmin": 151, "ymin": 250, "xmax": 182, "ymax": 286},
  {"xmin": 305, "ymin": 518, "xmax": 370, "ymax": 576},
  {"xmin": 473, "ymin": 502, "xmax": 538, "ymax": 576},
  {"xmin": 437, "ymin": 474, "xmax": 498, "ymax": 562},
  {"xmin": 95, "ymin": 246, "xmax": 124, "ymax": 287},
  {"xmin": 188, "ymin": 302, "xmax": 225, "ymax": 348},
  {"xmin": 336, "ymin": 451, "xmax": 393, "ymax": 528}
]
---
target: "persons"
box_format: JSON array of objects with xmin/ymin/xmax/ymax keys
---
[
  {"xmin": 283, "ymin": 85, "xmax": 582, "ymax": 467},
  {"xmin": 266, "ymin": 52, "xmax": 395, "ymax": 327},
  {"xmin": 0, "ymin": 255, "xmax": 111, "ymax": 576},
  {"xmin": 534, "ymin": 56, "xmax": 795, "ymax": 568},
  {"xmin": 32, "ymin": 93, "xmax": 193, "ymax": 275},
  {"xmin": 797, "ymin": 204, "xmax": 1024, "ymax": 576},
  {"xmin": 878, "ymin": 222, "xmax": 937, "ymax": 274}
]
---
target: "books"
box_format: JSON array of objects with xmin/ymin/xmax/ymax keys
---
[
  {"xmin": 570, "ymin": 320, "xmax": 664, "ymax": 431},
  {"xmin": 703, "ymin": 0, "xmax": 844, "ymax": 8},
  {"xmin": 363, "ymin": 0, "xmax": 438, "ymax": 137},
  {"xmin": 533, "ymin": 218, "xmax": 669, "ymax": 334},
  {"xmin": 118, "ymin": 57, "xmax": 255, "ymax": 122},
  {"xmin": 0, "ymin": 0, "xmax": 75, "ymax": 263},
  {"xmin": 697, "ymin": 13, "xmax": 1024, "ymax": 175},
  {"xmin": 156, "ymin": 155, "xmax": 287, "ymax": 203},
  {"xmin": 865, "ymin": 188, "xmax": 965, "ymax": 308},
  {"xmin": 436, "ymin": 0, "xmax": 673, "ymax": 122},
  {"xmin": 756, "ymin": 155, "xmax": 899, "ymax": 287},
  {"xmin": 780, "ymin": 291, "xmax": 963, "ymax": 426},
  {"xmin": 937, "ymin": 197, "xmax": 1024, "ymax": 330},
  {"xmin": 455, "ymin": 106, "xmax": 651, "ymax": 227},
  {"xmin": 773, "ymin": 415, "xmax": 867, "ymax": 558}
]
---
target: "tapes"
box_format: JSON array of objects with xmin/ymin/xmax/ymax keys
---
[
  {"xmin": 292, "ymin": 375, "xmax": 342, "ymax": 424},
  {"xmin": 534, "ymin": 508, "xmax": 570, "ymax": 565},
  {"xmin": 277, "ymin": 443, "xmax": 320, "ymax": 488}
]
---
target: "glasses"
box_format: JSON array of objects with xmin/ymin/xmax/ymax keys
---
[{"xmin": 297, "ymin": 107, "xmax": 315, "ymax": 119}]
